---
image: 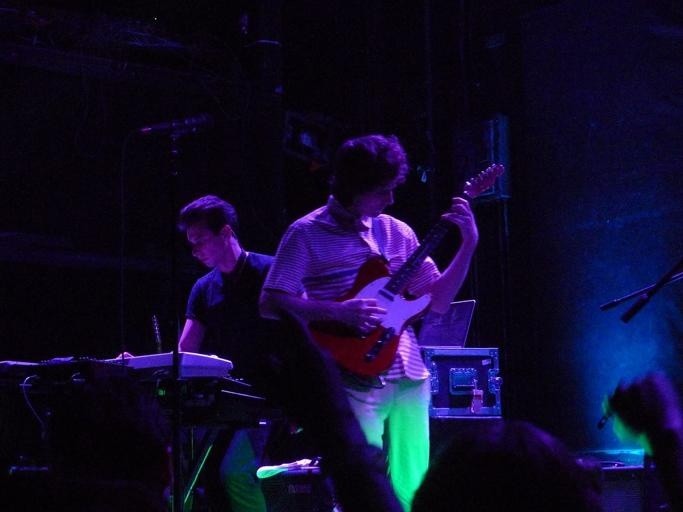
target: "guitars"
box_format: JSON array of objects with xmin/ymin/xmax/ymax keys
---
[{"xmin": 307, "ymin": 162, "xmax": 505, "ymax": 378}]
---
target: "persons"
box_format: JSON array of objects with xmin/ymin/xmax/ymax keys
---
[
  {"xmin": 170, "ymin": 191, "xmax": 326, "ymax": 511},
  {"xmin": 256, "ymin": 131, "xmax": 481, "ymax": 511},
  {"xmin": 408, "ymin": 370, "xmax": 680, "ymax": 510}
]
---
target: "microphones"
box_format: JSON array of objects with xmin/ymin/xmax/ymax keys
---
[{"xmin": 138, "ymin": 112, "xmax": 214, "ymax": 138}]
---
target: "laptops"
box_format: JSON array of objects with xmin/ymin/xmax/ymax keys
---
[{"xmin": 417, "ymin": 300, "xmax": 476, "ymax": 349}]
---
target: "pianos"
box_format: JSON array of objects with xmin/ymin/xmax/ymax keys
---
[{"xmin": 41, "ymin": 353, "xmax": 233, "ymax": 387}]
